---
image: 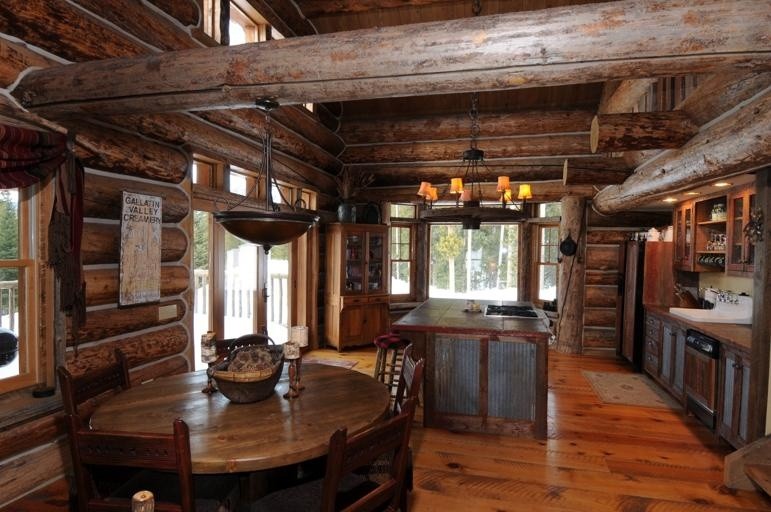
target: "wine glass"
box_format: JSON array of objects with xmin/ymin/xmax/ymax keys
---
[{"xmin": 706, "ymin": 231, "xmax": 725, "ymax": 251}]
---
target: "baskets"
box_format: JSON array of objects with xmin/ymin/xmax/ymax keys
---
[{"xmin": 207, "ymin": 344, "xmax": 283, "ymax": 403}]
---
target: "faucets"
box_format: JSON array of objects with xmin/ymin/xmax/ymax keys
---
[{"xmin": 710, "ymin": 287, "xmax": 726, "ymax": 303}]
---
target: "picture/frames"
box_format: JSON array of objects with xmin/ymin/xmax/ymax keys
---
[{"xmin": 118, "ymin": 190, "xmax": 163, "ymax": 307}]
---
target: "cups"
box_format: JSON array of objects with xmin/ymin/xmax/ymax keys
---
[{"xmin": 697, "ymin": 254, "xmax": 725, "ymax": 268}]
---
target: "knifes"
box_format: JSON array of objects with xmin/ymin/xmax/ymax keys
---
[{"xmin": 674, "ymin": 284, "xmax": 686, "ymax": 301}]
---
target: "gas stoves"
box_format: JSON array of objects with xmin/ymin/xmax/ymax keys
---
[{"xmin": 483, "ymin": 305, "xmax": 543, "ymax": 319}]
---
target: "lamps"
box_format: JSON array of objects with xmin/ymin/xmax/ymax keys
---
[
  {"xmin": 209, "ymin": 96, "xmax": 320, "ymax": 255},
  {"xmin": 411, "ymin": 92, "xmax": 533, "ymax": 229}
]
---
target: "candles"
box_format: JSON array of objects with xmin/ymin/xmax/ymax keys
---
[
  {"xmin": 201, "ymin": 331, "xmax": 218, "ymax": 364},
  {"xmin": 283, "ymin": 341, "xmax": 299, "ymax": 359},
  {"xmin": 292, "ymin": 325, "xmax": 309, "ymax": 348}
]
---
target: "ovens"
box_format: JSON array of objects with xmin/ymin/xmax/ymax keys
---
[{"xmin": 685, "ymin": 329, "xmax": 719, "ymax": 431}]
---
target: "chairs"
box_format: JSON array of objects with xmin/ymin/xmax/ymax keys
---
[
  {"xmin": 207, "ymin": 325, "xmax": 267, "ymax": 367},
  {"xmin": 65, "ymin": 411, "xmax": 241, "ymax": 511},
  {"xmin": 386, "ymin": 343, "xmax": 425, "ymax": 419},
  {"xmin": 53, "ymin": 348, "xmax": 132, "ymax": 512},
  {"xmin": 247, "ymin": 394, "xmax": 418, "ymax": 512}
]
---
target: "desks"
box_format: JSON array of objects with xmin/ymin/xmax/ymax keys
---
[{"xmin": 87, "ymin": 359, "xmax": 391, "ymax": 511}]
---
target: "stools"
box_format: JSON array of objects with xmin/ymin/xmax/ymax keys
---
[{"xmin": 372, "ymin": 333, "xmax": 409, "ymax": 402}]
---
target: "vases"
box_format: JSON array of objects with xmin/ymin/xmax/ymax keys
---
[{"xmin": 336, "ymin": 198, "xmax": 356, "ymax": 224}]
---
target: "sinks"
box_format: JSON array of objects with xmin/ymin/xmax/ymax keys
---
[{"xmin": 669, "ymin": 292, "xmax": 753, "ymax": 326}]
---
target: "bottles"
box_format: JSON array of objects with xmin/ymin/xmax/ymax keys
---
[{"xmin": 712, "ymin": 204, "xmax": 724, "ymax": 221}]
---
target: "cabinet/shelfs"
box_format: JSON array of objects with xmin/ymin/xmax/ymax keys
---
[
  {"xmin": 322, "ymin": 222, "xmax": 390, "ymax": 352},
  {"xmin": 618, "ymin": 181, "xmax": 771, "ymax": 451},
  {"xmin": 427, "ymin": 333, "xmax": 546, "ymax": 435}
]
